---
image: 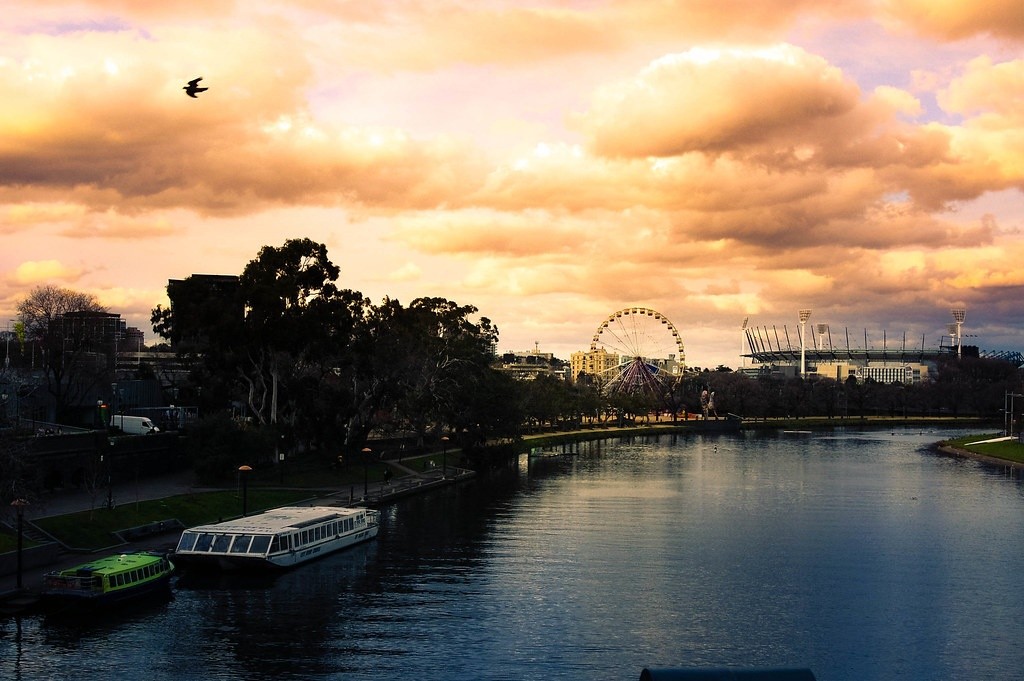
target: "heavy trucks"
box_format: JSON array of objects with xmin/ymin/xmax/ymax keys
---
[{"xmin": 94, "ymin": 402, "xmax": 199, "ymax": 435}]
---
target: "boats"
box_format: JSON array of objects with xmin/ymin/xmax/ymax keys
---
[
  {"xmin": 172, "ymin": 503, "xmax": 384, "ymax": 571},
  {"xmin": 41, "ymin": 551, "xmax": 175, "ymax": 611}
]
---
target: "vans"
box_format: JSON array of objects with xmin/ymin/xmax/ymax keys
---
[{"xmin": 110, "ymin": 414, "xmax": 160, "ymax": 435}]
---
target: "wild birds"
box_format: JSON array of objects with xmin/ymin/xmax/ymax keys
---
[{"xmin": 183, "ymin": 77, "xmax": 209, "ymax": 98}]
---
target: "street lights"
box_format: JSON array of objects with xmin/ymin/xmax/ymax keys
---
[
  {"xmin": 441, "ymin": 436, "xmax": 449, "ymax": 479},
  {"xmin": 110, "ymin": 382, "xmax": 117, "ymax": 436},
  {"xmin": 11, "ymin": 499, "xmax": 31, "ymax": 589},
  {"xmin": 237, "ymin": 464, "xmax": 253, "ymax": 519},
  {"xmin": 104, "ymin": 438, "xmax": 116, "ymax": 511},
  {"xmin": 361, "ymin": 447, "xmax": 372, "ymax": 501}
]
